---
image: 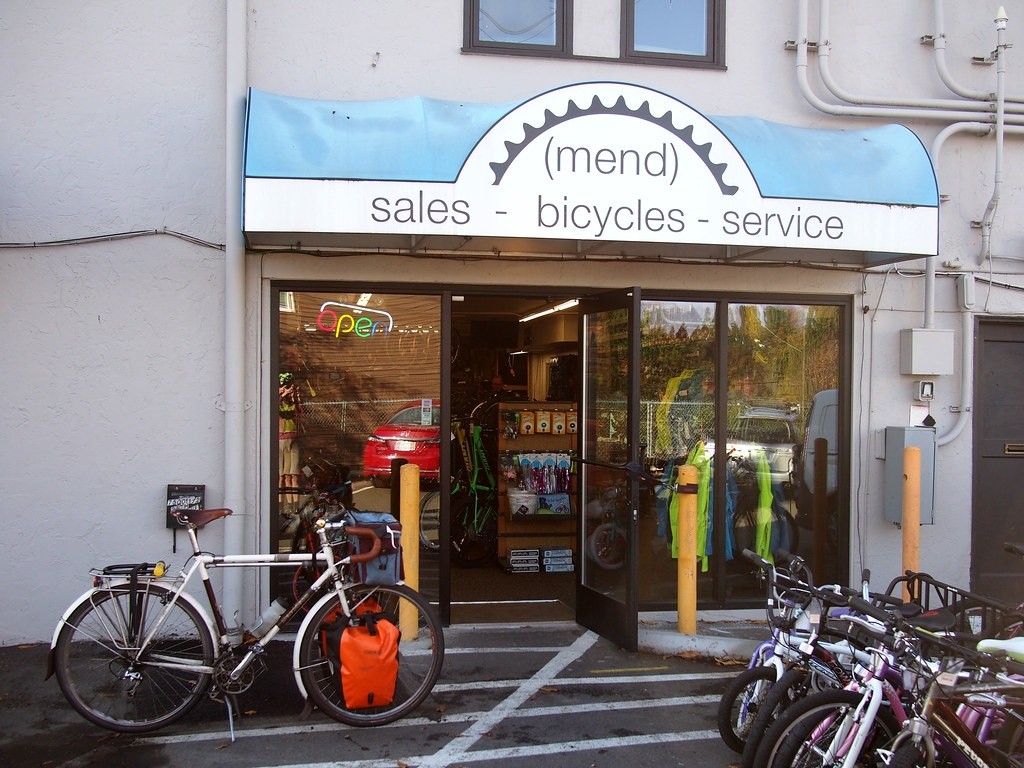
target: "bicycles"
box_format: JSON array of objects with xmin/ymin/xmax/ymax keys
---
[
  {"xmin": 712, "ymin": 549, "xmax": 1023, "ymax": 768},
  {"xmin": 47, "ymin": 488, "xmax": 445, "ymax": 748}
]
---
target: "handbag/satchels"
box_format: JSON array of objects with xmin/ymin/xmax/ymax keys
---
[
  {"xmin": 328, "ymin": 611, "xmax": 402, "ymax": 712},
  {"xmin": 320, "ymin": 595, "xmax": 383, "ymax": 688}
]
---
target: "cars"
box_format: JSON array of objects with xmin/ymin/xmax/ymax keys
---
[
  {"xmin": 589, "ymin": 383, "xmax": 843, "ymax": 574},
  {"xmin": 362, "ymin": 400, "xmax": 443, "ymax": 488}
]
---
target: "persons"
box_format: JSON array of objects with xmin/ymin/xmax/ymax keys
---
[{"xmin": 279, "ymin": 372, "xmax": 306, "ymax": 513}]
